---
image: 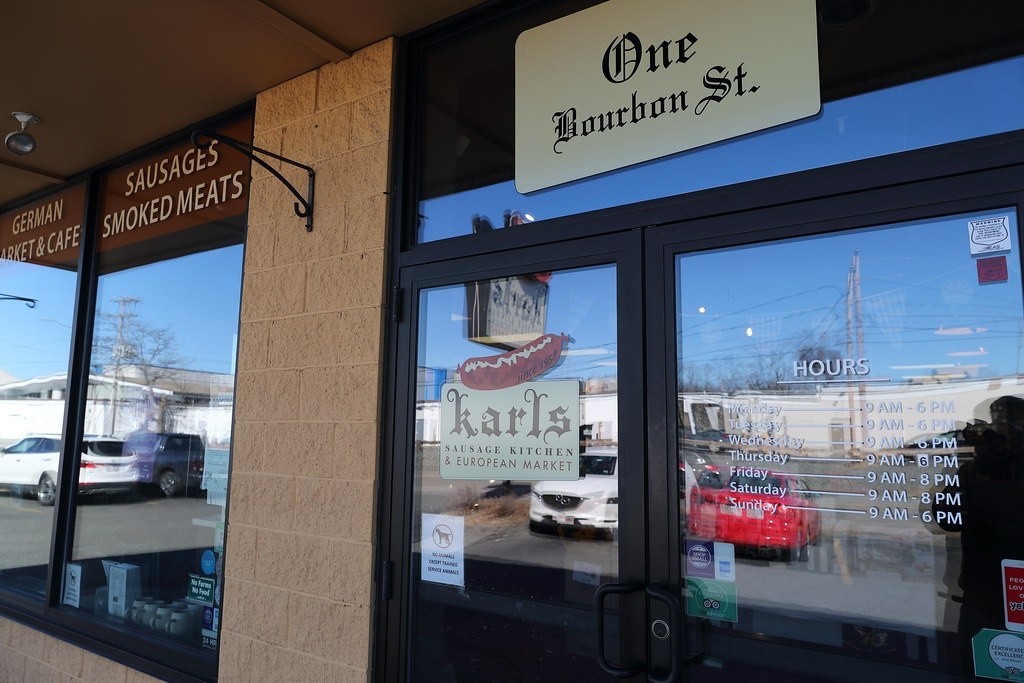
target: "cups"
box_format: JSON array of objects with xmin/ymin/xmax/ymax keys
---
[{"xmin": 123, "ymin": 597, "xmax": 203, "ymax": 641}]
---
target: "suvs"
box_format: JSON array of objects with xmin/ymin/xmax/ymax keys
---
[{"xmin": 124, "ymin": 430, "xmax": 207, "ymax": 498}]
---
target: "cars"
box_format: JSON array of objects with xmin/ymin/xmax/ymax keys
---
[
  {"xmin": 1, "ymin": 434, "xmax": 140, "ymax": 507},
  {"xmin": 690, "ymin": 466, "xmax": 822, "ymax": 563},
  {"xmin": 529, "ymin": 446, "xmax": 716, "ymax": 535},
  {"xmin": 683, "ymin": 428, "xmax": 739, "ymax": 453},
  {"xmin": 901, "ymin": 429, "xmax": 970, "ymax": 460}
]
